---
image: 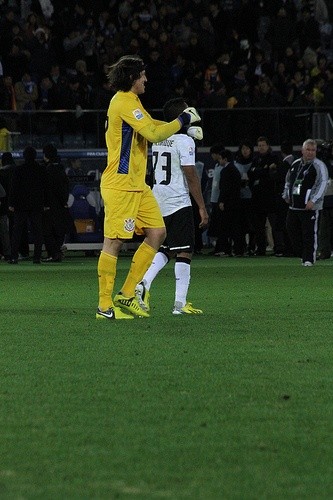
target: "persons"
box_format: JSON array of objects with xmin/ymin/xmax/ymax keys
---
[
  {"xmin": 134, "ymin": 97, "xmax": 209, "ymax": 315},
  {"xmin": 0, "ymin": 0, "xmax": 333, "ymax": 264},
  {"xmin": 95, "ymin": 55, "xmax": 203, "ymax": 320},
  {"xmin": 8, "ymin": 146, "xmax": 50, "ymax": 264},
  {"xmin": 247, "ymin": 136, "xmax": 284, "ymax": 257},
  {"xmin": 208, "ymin": 150, "xmax": 242, "ymax": 257},
  {"xmin": 281, "ymin": 139, "xmax": 329, "ymax": 267}
]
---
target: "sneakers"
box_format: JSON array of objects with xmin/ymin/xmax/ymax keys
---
[
  {"xmin": 173, "ymin": 302, "xmax": 203, "ymax": 315},
  {"xmin": 96, "ymin": 303, "xmax": 134, "ymax": 319},
  {"xmin": 136, "ymin": 281, "xmax": 150, "ymax": 312},
  {"xmin": 114, "ymin": 292, "xmax": 150, "ymax": 317}
]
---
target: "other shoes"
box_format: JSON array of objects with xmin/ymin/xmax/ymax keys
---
[
  {"xmin": 305, "ymin": 261, "xmax": 312, "ymax": 267},
  {"xmin": 33, "ymin": 256, "xmax": 62, "ymax": 264},
  {"xmin": 317, "ymin": 253, "xmax": 331, "ymax": 260},
  {"xmin": 209, "ymin": 249, "xmax": 266, "ymax": 257},
  {"xmin": 1, "ymin": 254, "xmax": 31, "ymax": 263}
]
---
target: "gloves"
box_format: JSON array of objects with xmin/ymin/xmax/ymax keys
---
[
  {"xmin": 178, "ymin": 107, "xmax": 201, "ymax": 127},
  {"xmin": 180, "ymin": 125, "xmax": 203, "ymax": 140}
]
